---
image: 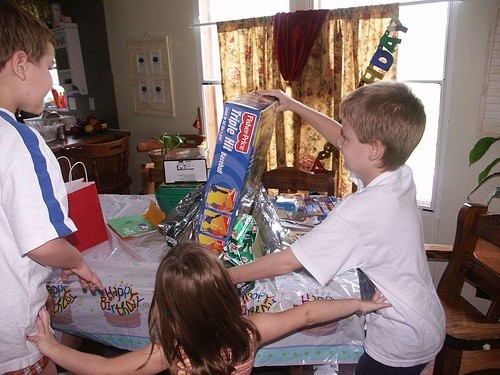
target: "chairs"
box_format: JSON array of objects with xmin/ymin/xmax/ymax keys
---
[
  {"xmin": 260, "ymin": 167, "xmax": 335, "ymax": 197},
  {"xmin": 60, "ymin": 132, "xmax": 130, "ymax": 194},
  {"xmin": 136, "ymin": 134, "xmax": 206, "ymax": 194},
  {"xmin": 420, "ymin": 202, "xmax": 500, "ymax": 375}
]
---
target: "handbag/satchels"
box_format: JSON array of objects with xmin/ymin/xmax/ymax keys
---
[
  {"xmin": 56, "ymin": 155, "xmax": 110, "ymax": 253},
  {"xmin": 156, "ymin": 183, "xmax": 207, "ymax": 249}
]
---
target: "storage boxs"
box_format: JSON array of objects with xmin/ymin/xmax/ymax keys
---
[
  {"xmin": 154, "ymin": 147, "xmax": 206, "ymax": 217},
  {"xmin": 43, "ymin": 4, "xmax": 61, "ymax": 30},
  {"xmin": 197, "ymin": 94, "xmax": 279, "ymax": 256}
]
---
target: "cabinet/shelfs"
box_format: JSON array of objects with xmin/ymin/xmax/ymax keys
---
[{"xmin": 49, "ymin": 22, "xmax": 88, "ymax": 95}]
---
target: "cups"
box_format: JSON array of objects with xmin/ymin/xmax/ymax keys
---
[{"xmin": 23, "ymin": 118, "xmax": 44, "ymax": 138}]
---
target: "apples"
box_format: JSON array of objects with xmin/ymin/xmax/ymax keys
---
[{"xmin": 70, "ymin": 116, "xmax": 107, "ymax": 134}]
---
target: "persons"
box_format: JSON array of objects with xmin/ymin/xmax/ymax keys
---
[
  {"xmin": 26, "ymin": 241, "xmax": 392, "ymax": 375},
  {"xmin": 226, "ymin": 83, "xmax": 446, "ymax": 375},
  {"xmin": 0, "ymin": 0, "xmax": 105, "ymax": 375}
]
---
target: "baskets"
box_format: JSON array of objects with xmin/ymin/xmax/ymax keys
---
[{"xmin": 23, "ymin": 114, "xmax": 77, "ymax": 142}]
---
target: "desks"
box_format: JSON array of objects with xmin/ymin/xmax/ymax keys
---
[{"xmin": 42, "ymin": 193, "xmax": 364, "ymax": 375}]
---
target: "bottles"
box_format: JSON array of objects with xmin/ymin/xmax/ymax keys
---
[
  {"xmin": 161, "ymin": 136, "xmax": 173, "ymax": 154},
  {"xmin": 57, "ymin": 124, "xmax": 66, "ymax": 140}
]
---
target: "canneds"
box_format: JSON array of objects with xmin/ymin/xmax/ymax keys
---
[{"xmin": 56, "ymin": 124, "xmax": 66, "ymax": 139}]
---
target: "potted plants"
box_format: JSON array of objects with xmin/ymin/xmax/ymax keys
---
[{"xmin": 147, "ymin": 133, "xmax": 184, "ymax": 167}]
